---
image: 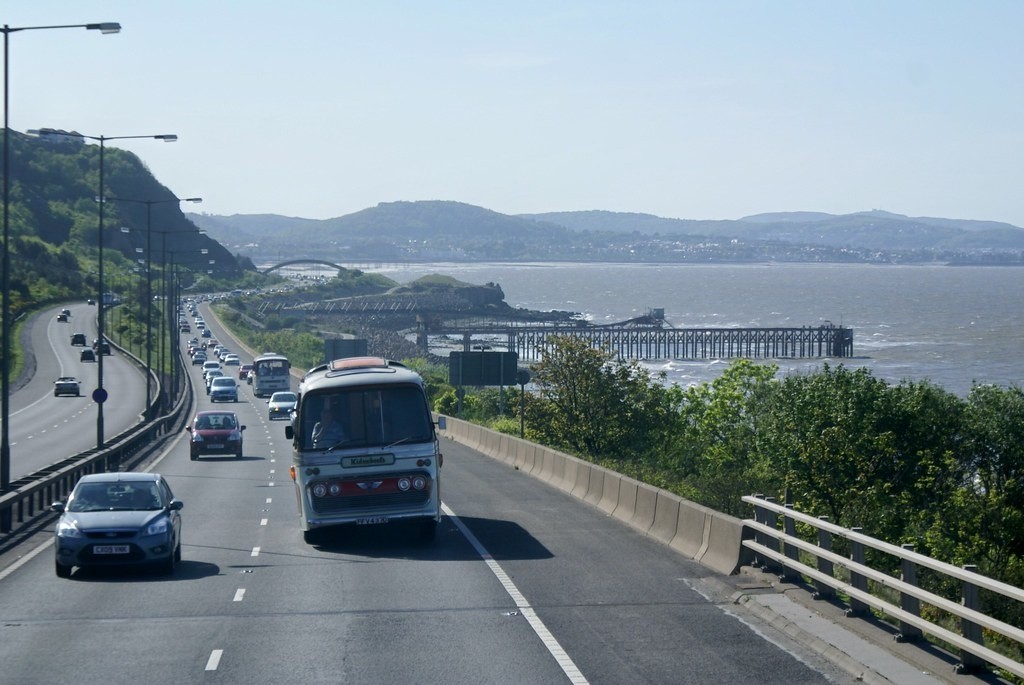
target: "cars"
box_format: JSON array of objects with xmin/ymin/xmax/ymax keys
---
[
  {"xmin": 186, "ymin": 410, "xmax": 246, "ymax": 460},
  {"xmin": 51, "ymin": 472, "xmax": 184, "ymax": 577},
  {"xmin": 195, "ymin": 318, "xmax": 240, "ymax": 366},
  {"xmin": 246, "ymin": 284, "xmax": 294, "ymax": 296},
  {"xmin": 195, "ymin": 294, "xmax": 225, "ymax": 304},
  {"xmin": 52, "ymin": 377, "xmax": 81, "ymax": 397},
  {"xmin": 70, "ymin": 333, "xmax": 111, "ymax": 362},
  {"xmin": 187, "ymin": 340, "xmax": 208, "ymax": 366},
  {"xmin": 210, "ymin": 377, "xmax": 240, "ymax": 403},
  {"xmin": 177, "ymin": 299, "xmax": 191, "ymax": 334},
  {"xmin": 247, "ymin": 370, "xmax": 253, "ymax": 384},
  {"xmin": 238, "ymin": 365, "xmax": 253, "ymax": 379},
  {"xmin": 56, "ymin": 298, "xmax": 95, "ymax": 322},
  {"xmin": 201, "ymin": 361, "xmax": 223, "ymax": 395},
  {"xmin": 186, "ymin": 299, "xmax": 198, "ymax": 316},
  {"xmin": 266, "ymin": 392, "xmax": 298, "ymax": 421}
]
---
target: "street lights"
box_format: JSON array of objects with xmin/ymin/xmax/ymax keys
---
[
  {"xmin": 120, "ymin": 227, "xmax": 208, "ymax": 416},
  {"xmin": 135, "ymin": 247, "xmax": 215, "ymax": 416},
  {"xmin": 0, "ymin": 22, "xmax": 122, "ymax": 532},
  {"xmin": 25, "ymin": 130, "xmax": 178, "ymax": 449},
  {"xmin": 94, "ymin": 195, "xmax": 203, "ymax": 422}
]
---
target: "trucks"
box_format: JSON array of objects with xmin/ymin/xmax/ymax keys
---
[{"xmin": 103, "ymin": 293, "xmax": 113, "ymax": 305}]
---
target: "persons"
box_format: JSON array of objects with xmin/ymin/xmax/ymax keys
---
[
  {"xmin": 258, "ymin": 364, "xmax": 268, "ymax": 377},
  {"xmin": 311, "ymin": 408, "xmax": 346, "ymax": 449}
]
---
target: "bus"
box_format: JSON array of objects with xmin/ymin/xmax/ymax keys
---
[
  {"xmin": 252, "ymin": 353, "xmax": 292, "ymax": 398},
  {"xmin": 285, "ymin": 356, "xmax": 446, "ymax": 544}
]
